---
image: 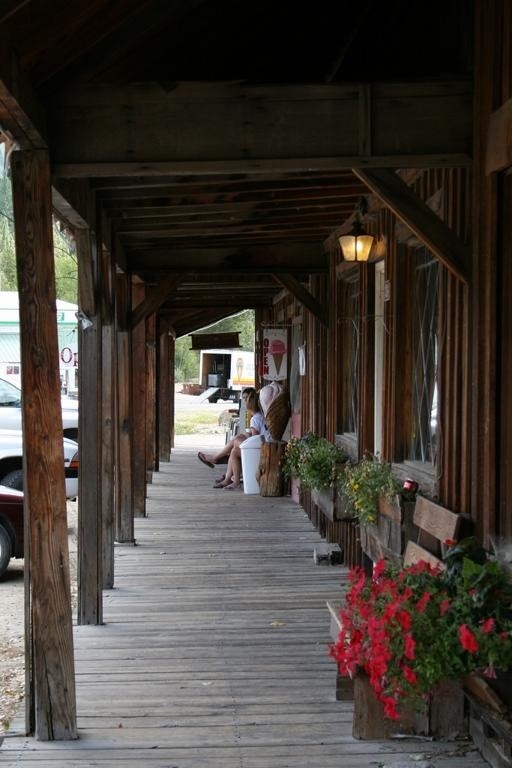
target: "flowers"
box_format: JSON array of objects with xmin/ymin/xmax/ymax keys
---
[{"xmin": 326, "ymin": 538, "xmax": 512, "ymax": 721}]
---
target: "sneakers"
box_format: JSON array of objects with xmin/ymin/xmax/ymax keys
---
[{"xmin": 215, "ymin": 474, "xmax": 225, "ymax": 482}]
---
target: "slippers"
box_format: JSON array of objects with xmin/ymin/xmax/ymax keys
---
[
  {"xmin": 198, "ymin": 452, "xmax": 215, "ymax": 468},
  {"xmin": 222, "ymin": 484, "xmax": 241, "ymax": 490},
  {"xmin": 213, "ymin": 480, "xmax": 231, "ymax": 488}
]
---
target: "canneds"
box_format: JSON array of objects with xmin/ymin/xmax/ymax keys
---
[{"xmin": 403, "ymin": 479, "xmax": 418, "ymax": 494}]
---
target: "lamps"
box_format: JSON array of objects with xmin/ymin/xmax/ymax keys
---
[{"xmin": 338, "ymin": 213, "xmax": 374, "ymax": 262}]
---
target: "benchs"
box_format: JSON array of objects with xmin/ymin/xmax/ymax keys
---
[{"xmin": 326, "ymin": 494, "xmax": 474, "ymax": 740}]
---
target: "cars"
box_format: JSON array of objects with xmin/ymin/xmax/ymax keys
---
[{"xmin": 1, "ymin": 371, "xmax": 80, "ymax": 579}]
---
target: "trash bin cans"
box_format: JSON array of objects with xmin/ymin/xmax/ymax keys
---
[{"xmin": 240, "ymin": 434, "xmax": 264, "ymax": 495}]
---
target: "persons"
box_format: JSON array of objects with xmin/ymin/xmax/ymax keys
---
[{"xmin": 196, "ymin": 387, "xmax": 271, "ymax": 491}]
